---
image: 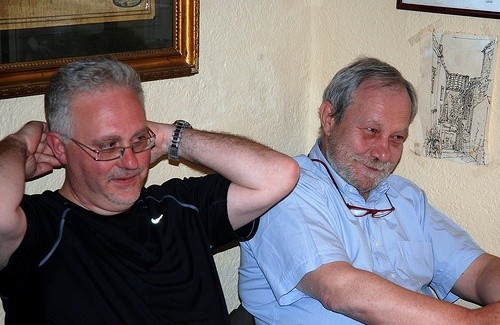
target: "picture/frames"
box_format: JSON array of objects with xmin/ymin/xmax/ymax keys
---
[{"xmin": 0, "ymin": 0, "xmax": 199, "ymax": 99}]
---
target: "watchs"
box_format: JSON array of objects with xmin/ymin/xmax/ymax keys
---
[{"xmin": 167, "ymin": 120, "xmax": 193, "ymax": 161}]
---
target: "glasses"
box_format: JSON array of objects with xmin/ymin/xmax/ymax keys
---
[
  {"xmin": 311, "ymin": 158, "xmax": 396, "ymax": 218},
  {"xmin": 58, "ymin": 127, "xmax": 157, "ymax": 161}
]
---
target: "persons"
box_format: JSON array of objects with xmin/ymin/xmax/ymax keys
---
[
  {"xmin": 0, "ymin": 57, "xmax": 300, "ymax": 325},
  {"xmin": 238, "ymin": 58, "xmax": 500, "ymax": 325}
]
